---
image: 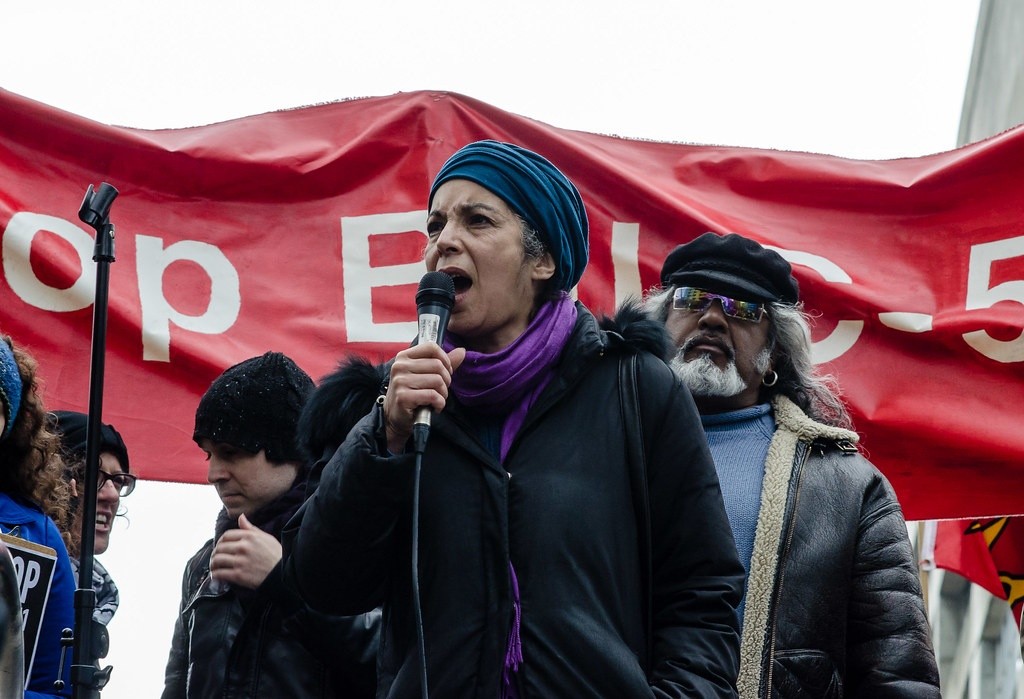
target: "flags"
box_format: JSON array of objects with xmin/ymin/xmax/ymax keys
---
[{"xmin": 919, "ymin": 516, "xmax": 1024, "ymax": 663}]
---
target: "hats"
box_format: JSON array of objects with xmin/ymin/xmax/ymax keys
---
[
  {"xmin": 193, "ymin": 351, "xmax": 317, "ymax": 453},
  {"xmin": 660, "ymin": 232, "xmax": 801, "ymax": 305},
  {"xmin": 427, "ymin": 139, "xmax": 590, "ymax": 292},
  {"xmin": 44, "ymin": 410, "xmax": 128, "ymax": 473}
]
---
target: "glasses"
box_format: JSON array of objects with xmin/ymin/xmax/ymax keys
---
[
  {"xmin": 61, "ymin": 468, "xmax": 137, "ymax": 496},
  {"xmin": 671, "ymin": 284, "xmax": 770, "ymax": 323}
]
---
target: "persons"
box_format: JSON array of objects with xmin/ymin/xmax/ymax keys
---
[
  {"xmin": 280, "ymin": 140, "xmax": 746, "ymax": 698},
  {"xmin": 639, "ymin": 231, "xmax": 940, "ymax": 699},
  {"xmin": 160, "ymin": 351, "xmax": 382, "ymax": 699},
  {"xmin": 0, "ymin": 335, "xmax": 76, "ymax": 699},
  {"xmin": 42, "ymin": 411, "xmax": 138, "ymax": 626}
]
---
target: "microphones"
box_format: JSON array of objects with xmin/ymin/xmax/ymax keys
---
[{"xmin": 411, "ymin": 271, "xmax": 456, "ymax": 454}]
